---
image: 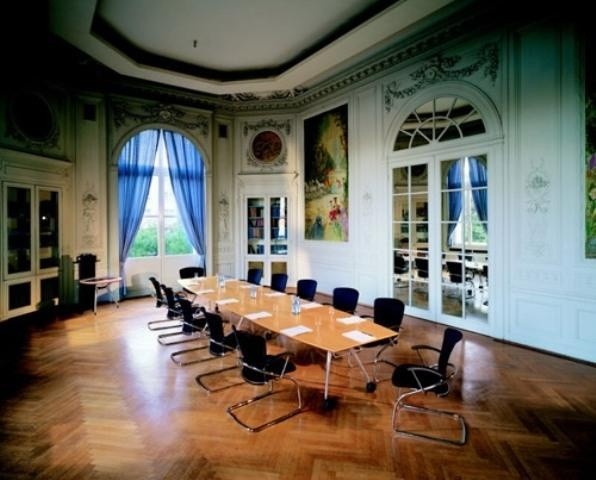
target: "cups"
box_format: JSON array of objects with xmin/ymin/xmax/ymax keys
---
[
  {"xmin": 292, "ymin": 297, "xmax": 300, "ymax": 314},
  {"xmin": 251, "ymin": 287, "xmax": 257, "ymax": 298},
  {"xmin": 220, "ymin": 278, "xmax": 224, "ymax": 288}
]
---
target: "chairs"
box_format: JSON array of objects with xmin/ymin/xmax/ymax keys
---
[
  {"xmin": 286, "ymin": 279, "xmax": 318, "ymax": 301},
  {"xmin": 179, "ymin": 267, "xmax": 205, "ymax": 279},
  {"xmin": 171, "ymin": 293, "xmax": 231, "ymax": 366},
  {"xmin": 158, "ymin": 283, "xmax": 209, "ymax": 344},
  {"xmin": 196, "ymin": 305, "xmax": 250, "ymax": 393},
  {"xmin": 348, "ymin": 298, "xmax": 403, "ymax": 384},
  {"xmin": 245, "ymin": 268, "xmax": 262, "ymax": 286},
  {"xmin": 149, "ymin": 275, "xmax": 196, "ymax": 329},
  {"xmin": 393, "ymin": 245, "xmax": 490, "ymax": 311},
  {"xmin": 318, "ymin": 287, "xmax": 362, "ymax": 359},
  {"xmin": 270, "ymin": 272, "xmax": 287, "ymax": 293},
  {"xmin": 390, "ymin": 328, "xmax": 467, "ymax": 446},
  {"xmin": 227, "ymin": 325, "xmax": 309, "ymax": 432}
]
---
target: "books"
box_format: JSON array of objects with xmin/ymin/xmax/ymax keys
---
[{"xmin": 247, "ymin": 197, "xmax": 287, "ymax": 255}]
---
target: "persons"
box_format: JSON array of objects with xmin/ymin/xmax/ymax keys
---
[{"xmin": 308, "ymin": 197, "xmax": 348, "ymax": 241}]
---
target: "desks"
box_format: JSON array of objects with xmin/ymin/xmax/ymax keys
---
[
  {"xmin": 80, "ymin": 276, "xmax": 123, "ymax": 315},
  {"xmin": 175, "ymin": 275, "xmax": 400, "ymax": 407}
]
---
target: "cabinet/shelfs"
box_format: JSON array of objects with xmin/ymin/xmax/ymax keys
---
[
  {"xmin": 0, "ymin": 163, "xmax": 66, "ymax": 323},
  {"xmin": 237, "ymin": 175, "xmax": 299, "ymax": 286}
]
